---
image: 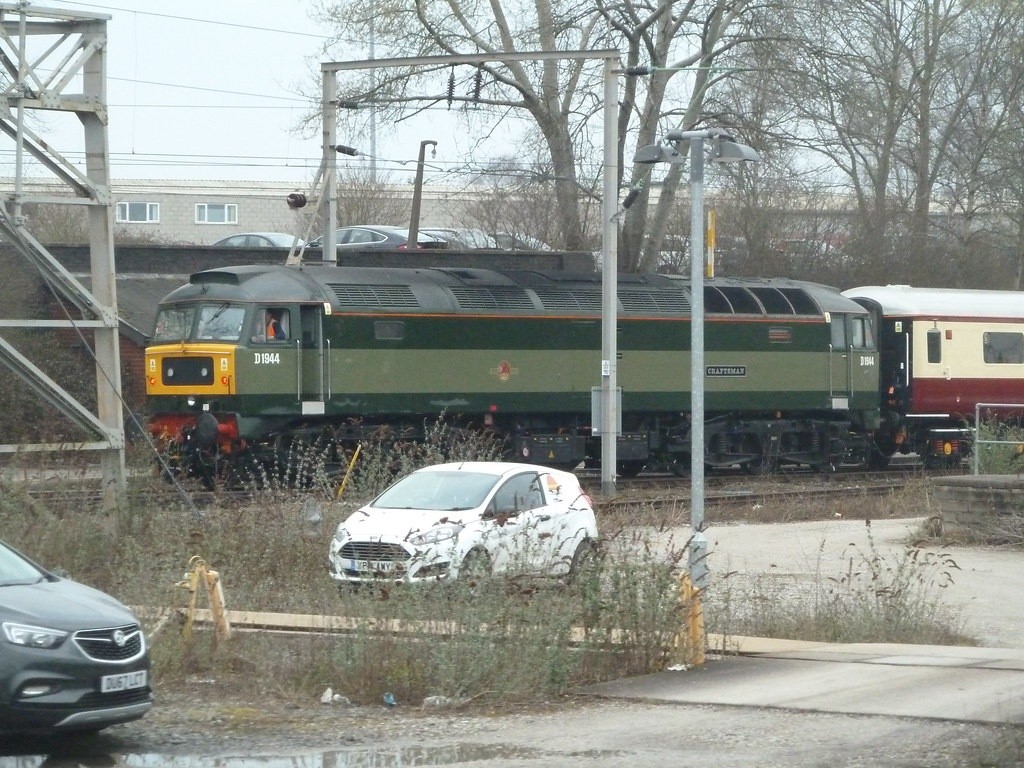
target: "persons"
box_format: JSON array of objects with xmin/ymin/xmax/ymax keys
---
[{"xmin": 253, "ymin": 308, "xmax": 286, "ymax": 340}]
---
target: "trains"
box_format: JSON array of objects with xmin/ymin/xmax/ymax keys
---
[{"xmin": 143, "ymin": 167, "xmax": 1024, "ymax": 491}]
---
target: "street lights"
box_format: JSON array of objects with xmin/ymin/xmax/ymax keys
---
[
  {"xmin": 633, "ymin": 128, "xmax": 761, "ymax": 591},
  {"xmin": 407, "ymin": 140, "xmax": 439, "ymax": 249}
]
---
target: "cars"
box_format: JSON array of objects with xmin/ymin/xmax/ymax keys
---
[
  {"xmin": 0, "ymin": 538, "xmax": 154, "ymax": 747},
  {"xmin": 324, "ymin": 462, "xmax": 604, "ymax": 590},
  {"xmin": 762, "ymin": 236, "xmax": 863, "ymax": 270},
  {"xmin": 211, "ymin": 232, "xmax": 311, "ymax": 249},
  {"xmin": 591, "ymin": 229, "xmax": 693, "ymax": 274},
  {"xmin": 307, "ymin": 224, "xmax": 553, "ymax": 254}
]
